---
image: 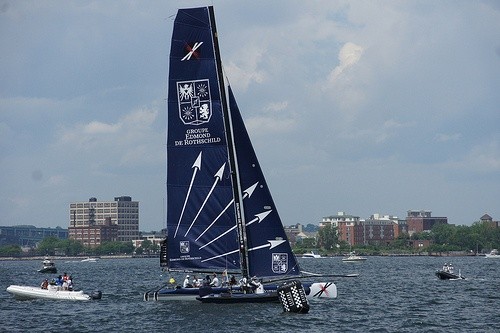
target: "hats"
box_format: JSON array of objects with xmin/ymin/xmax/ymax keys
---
[
  {"xmin": 213, "ymin": 274, "xmax": 216, "ymax": 276},
  {"xmin": 450, "ymin": 264, "xmax": 452, "ymax": 265},
  {"xmin": 186, "ymin": 275, "xmax": 190, "ymax": 277},
  {"xmin": 444, "ymin": 263, "xmax": 447, "ymax": 265}
]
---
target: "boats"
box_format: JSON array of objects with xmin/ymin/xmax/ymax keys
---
[
  {"xmin": 6, "ymin": 283, "xmax": 91, "ymax": 303},
  {"xmin": 341, "ymin": 254, "xmax": 368, "ymax": 262},
  {"xmin": 36, "ymin": 260, "xmax": 57, "ymax": 274},
  {"xmin": 301, "ymin": 251, "xmax": 322, "ymax": 259},
  {"xmin": 81, "ymin": 258, "xmax": 97, "ymax": 263},
  {"xmin": 434, "ymin": 269, "xmax": 465, "ymax": 281},
  {"xmin": 484, "ymin": 250, "xmax": 500, "ymax": 259}
]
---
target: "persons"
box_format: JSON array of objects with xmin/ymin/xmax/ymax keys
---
[
  {"xmin": 183, "ymin": 271, "xmax": 265, "ymax": 295},
  {"xmin": 40, "ymin": 272, "xmax": 74, "ymax": 292},
  {"xmin": 442, "ymin": 263, "xmax": 454, "ymax": 275}
]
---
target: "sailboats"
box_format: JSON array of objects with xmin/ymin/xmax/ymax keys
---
[{"xmin": 143, "ymin": 5, "xmax": 361, "ymax": 303}]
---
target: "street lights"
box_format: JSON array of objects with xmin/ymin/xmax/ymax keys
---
[{"xmin": 477, "ymin": 239, "xmax": 479, "ymax": 255}]
